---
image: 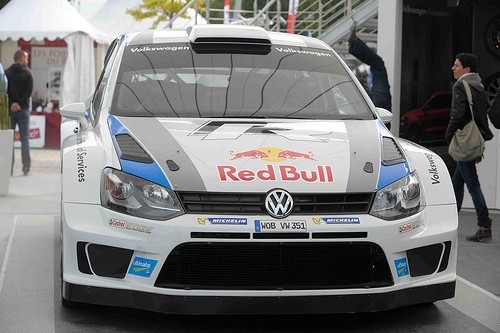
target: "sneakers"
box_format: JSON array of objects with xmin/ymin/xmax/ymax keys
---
[{"xmin": 466, "ymin": 228, "xmax": 492, "ymax": 241}]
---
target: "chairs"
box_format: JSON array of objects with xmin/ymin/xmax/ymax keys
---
[
  {"xmin": 121, "ymin": 81, "xmax": 207, "ymax": 116},
  {"xmin": 227, "ymin": 70, "xmax": 325, "ymax": 115}
]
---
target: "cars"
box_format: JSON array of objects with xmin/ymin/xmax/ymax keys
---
[
  {"xmin": 400, "ymin": 89, "xmax": 452, "ymax": 141},
  {"xmin": 60, "ymin": 23, "xmax": 459, "ymax": 312}
]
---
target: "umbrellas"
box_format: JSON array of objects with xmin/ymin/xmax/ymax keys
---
[{"xmin": 0, "ymin": 0, "xmax": 104, "ymax": 50}]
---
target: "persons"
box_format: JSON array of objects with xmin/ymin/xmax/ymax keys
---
[
  {"xmin": 445, "ymin": 53, "xmax": 495, "ymax": 243},
  {"xmin": 3, "ymin": 49, "xmax": 34, "ymax": 178},
  {"xmin": 347, "ymin": 19, "xmax": 391, "ymax": 133},
  {"xmin": 28, "ymin": 90, "xmax": 41, "ymax": 113}
]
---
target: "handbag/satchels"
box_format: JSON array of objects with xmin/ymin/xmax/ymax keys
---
[{"xmin": 448, "ymin": 120, "xmax": 485, "ymax": 161}]
